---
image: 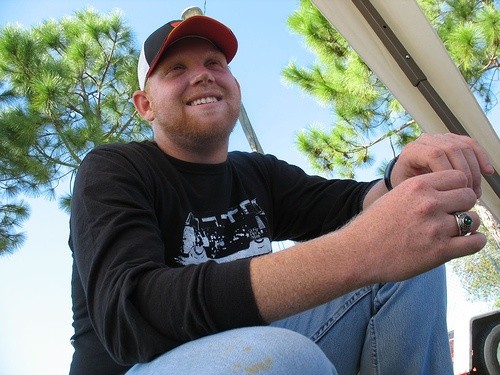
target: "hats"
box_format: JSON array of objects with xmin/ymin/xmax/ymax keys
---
[{"xmin": 138, "ymin": 15, "xmax": 238, "ymax": 92}]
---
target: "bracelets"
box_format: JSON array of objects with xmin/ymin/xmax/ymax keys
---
[{"xmin": 385, "ymin": 154, "xmax": 398, "ymax": 191}]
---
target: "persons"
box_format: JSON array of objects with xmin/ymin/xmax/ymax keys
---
[{"xmin": 67, "ymin": 15, "xmax": 495, "ymax": 375}]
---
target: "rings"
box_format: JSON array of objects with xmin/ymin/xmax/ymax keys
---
[{"xmin": 453, "ymin": 211, "xmax": 473, "ymax": 237}]
